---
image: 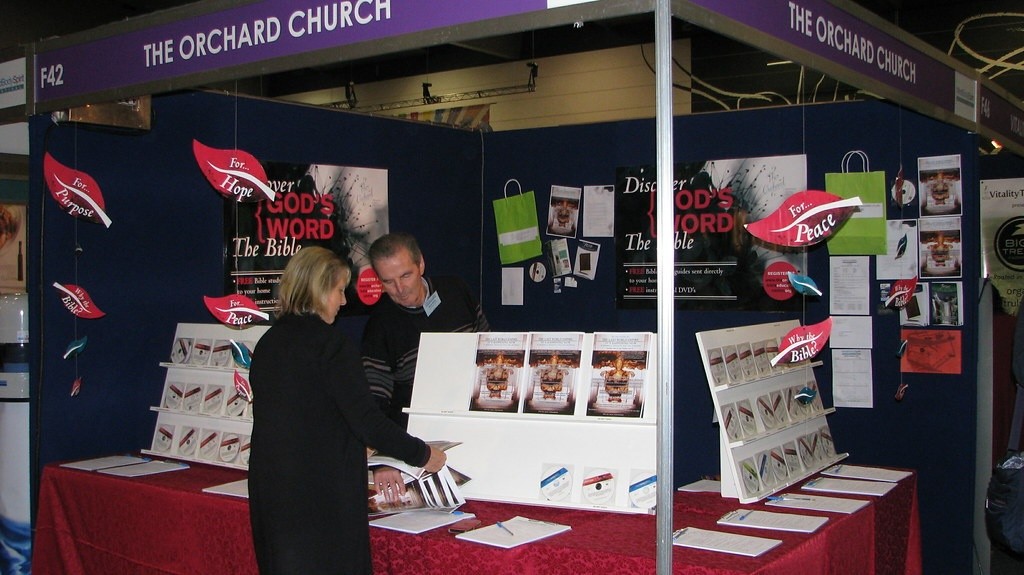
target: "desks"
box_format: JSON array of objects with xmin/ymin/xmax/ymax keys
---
[{"xmin": 31, "ymin": 451, "xmax": 921, "ymax": 575}]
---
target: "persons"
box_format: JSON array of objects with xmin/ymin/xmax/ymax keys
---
[
  {"xmin": 363, "ymin": 230, "xmax": 489, "ymax": 503},
  {"xmin": 248, "ymin": 247, "xmax": 446, "ymax": 575}
]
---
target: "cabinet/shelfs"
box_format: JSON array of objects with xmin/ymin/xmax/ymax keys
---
[
  {"xmin": 695, "ymin": 319, "xmax": 850, "ymax": 503},
  {"xmin": 402, "ymin": 332, "xmax": 658, "ymax": 516},
  {"xmin": 141, "ymin": 323, "xmax": 273, "ymax": 471}
]
